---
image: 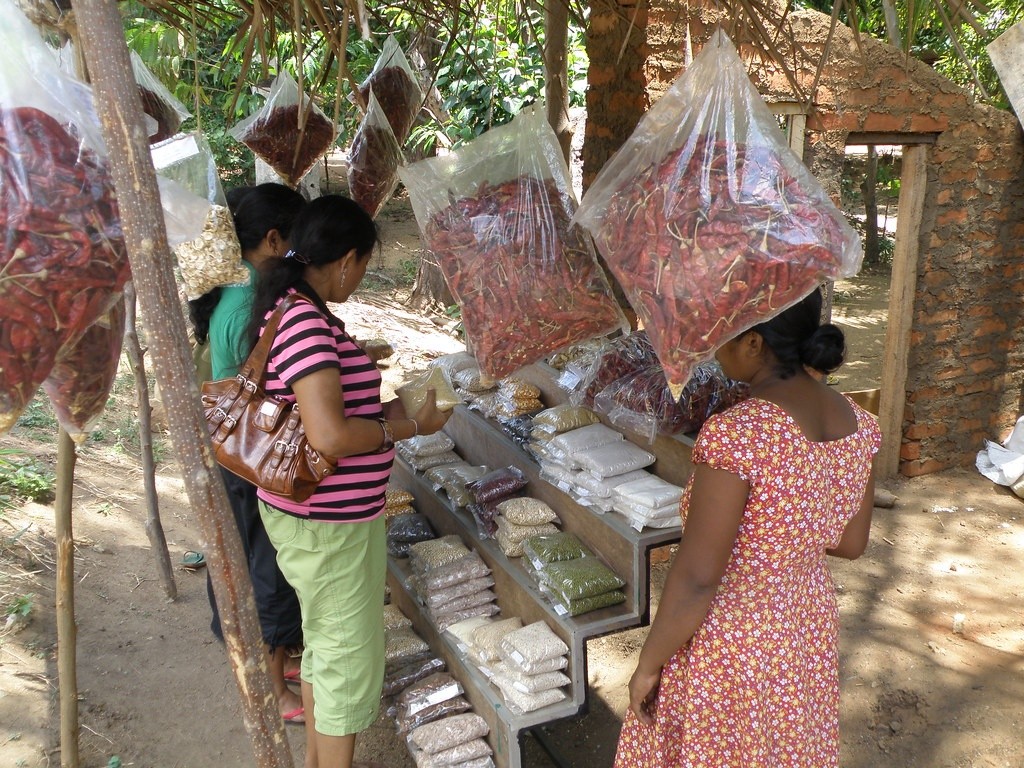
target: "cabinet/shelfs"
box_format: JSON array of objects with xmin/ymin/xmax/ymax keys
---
[{"xmin": 385, "ymin": 353, "xmax": 697, "ymax": 768}]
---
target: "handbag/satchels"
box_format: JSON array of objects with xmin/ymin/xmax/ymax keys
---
[{"xmin": 200, "ymin": 295, "xmax": 338, "ymax": 504}]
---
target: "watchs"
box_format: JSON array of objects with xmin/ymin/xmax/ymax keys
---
[{"xmin": 373, "ymin": 418, "xmax": 394, "ymax": 455}]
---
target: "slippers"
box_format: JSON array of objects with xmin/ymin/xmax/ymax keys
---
[
  {"xmin": 283, "ymin": 664, "xmax": 300, "ymax": 684},
  {"xmin": 179, "ymin": 550, "xmax": 206, "ymax": 568},
  {"xmin": 281, "ymin": 700, "xmax": 305, "ymax": 725}
]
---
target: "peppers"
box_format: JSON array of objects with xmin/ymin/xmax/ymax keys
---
[{"xmin": 0, "ymin": 65, "xmax": 849, "ymax": 448}]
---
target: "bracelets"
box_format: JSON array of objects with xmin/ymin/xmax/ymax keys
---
[{"xmin": 409, "ymin": 419, "xmax": 417, "ymax": 437}]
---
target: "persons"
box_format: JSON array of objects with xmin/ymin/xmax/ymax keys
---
[
  {"xmin": 188, "ymin": 184, "xmax": 395, "ymax": 724},
  {"xmin": 247, "ymin": 194, "xmax": 453, "ymax": 768},
  {"xmin": 613, "ymin": 286, "xmax": 882, "ymax": 768}
]
